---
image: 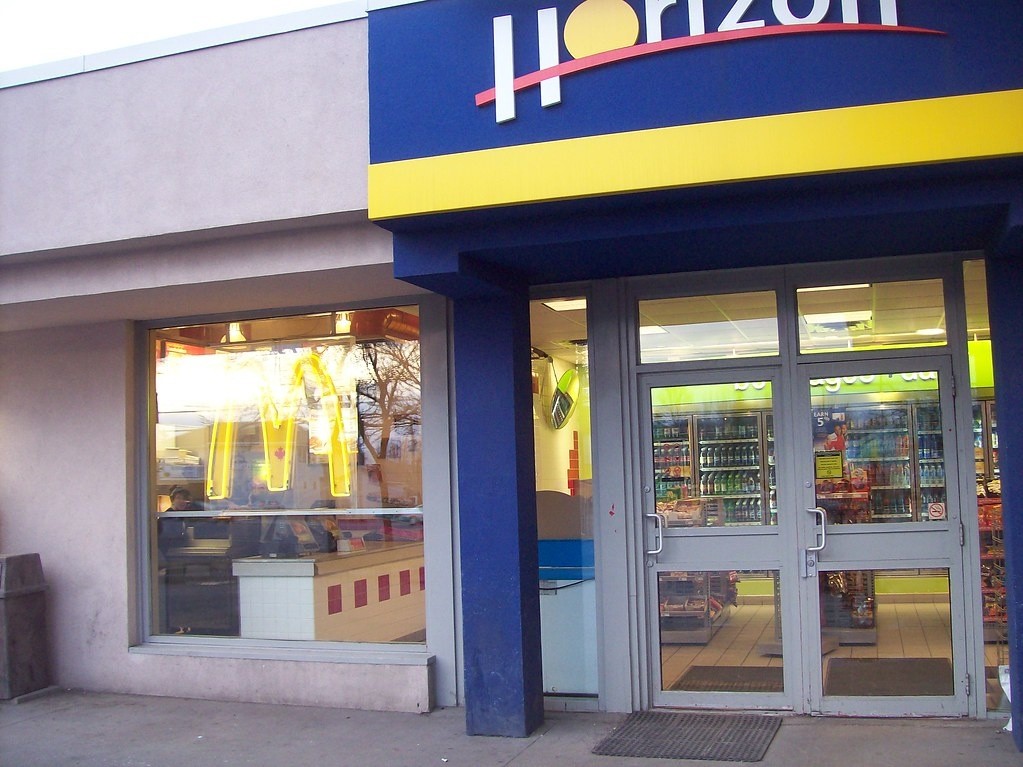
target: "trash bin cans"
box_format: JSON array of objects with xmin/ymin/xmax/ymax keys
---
[{"xmin": 0, "ymin": 552, "xmax": 50, "ymax": 700}]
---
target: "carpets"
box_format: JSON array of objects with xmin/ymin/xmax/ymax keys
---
[
  {"xmin": 670, "ymin": 665, "xmax": 784, "ymax": 693},
  {"xmin": 825, "ymin": 658, "xmax": 955, "ymax": 696},
  {"xmin": 592, "ymin": 710, "xmax": 783, "ymax": 763}
]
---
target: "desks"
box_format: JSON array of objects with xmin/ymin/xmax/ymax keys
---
[{"xmin": 162, "ymin": 538, "xmax": 236, "ymax": 636}]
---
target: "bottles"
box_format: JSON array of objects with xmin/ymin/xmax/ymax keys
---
[
  {"xmin": 814, "ymin": 406, "xmax": 948, "ymax": 526},
  {"xmin": 649, "ymin": 414, "xmax": 781, "ymax": 527},
  {"xmin": 972, "ymin": 407, "xmax": 1002, "ymax": 500}
]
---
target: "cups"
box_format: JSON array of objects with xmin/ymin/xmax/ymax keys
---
[{"xmin": 186, "ymin": 526, "xmax": 194, "ymax": 542}]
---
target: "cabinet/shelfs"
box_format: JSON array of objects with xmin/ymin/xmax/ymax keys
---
[
  {"xmin": 773, "ymin": 491, "xmax": 879, "ymax": 644},
  {"xmin": 655, "ymin": 498, "xmax": 729, "ymax": 644}
]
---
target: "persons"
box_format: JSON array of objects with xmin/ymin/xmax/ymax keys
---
[
  {"xmin": 824, "ymin": 424, "xmax": 847, "ymax": 451},
  {"xmin": 158, "ymin": 487, "xmax": 190, "ymax": 555}
]
